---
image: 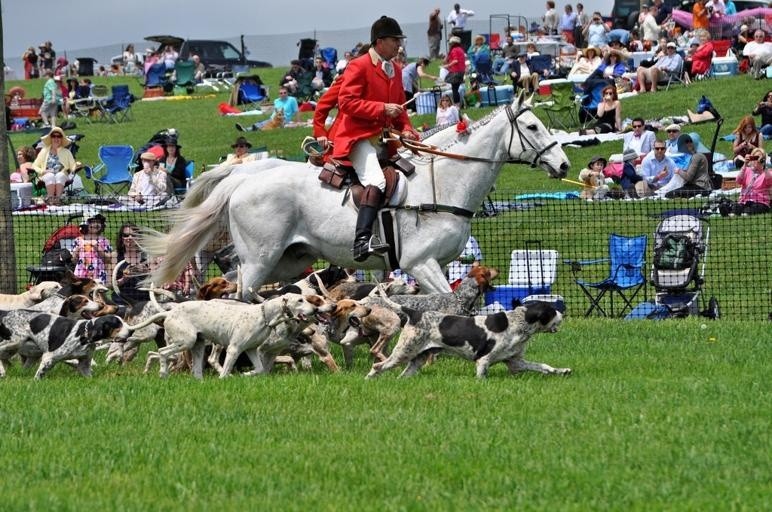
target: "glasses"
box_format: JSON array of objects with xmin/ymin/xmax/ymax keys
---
[
  {"xmin": 665, "ymin": 124, "xmax": 680, "ymax": 131},
  {"xmin": 279, "ymin": 92, "xmax": 287, "ymax": 94},
  {"xmin": 50, "ymin": 135, "xmax": 63, "ymax": 138},
  {"xmin": 755, "ymin": 35, "xmax": 764, "ymax": 38},
  {"xmin": 441, "ymin": 100, "xmax": 450, "ymax": 102},
  {"xmin": 766, "ymin": 95, "xmax": 772, "ymax": 97},
  {"xmin": 604, "ymin": 92, "xmax": 613, "ymax": 96},
  {"xmin": 610, "ymin": 54, "xmax": 617, "ymax": 57},
  {"xmin": 667, "ymin": 47, "xmax": 674, "ymax": 49},
  {"xmin": 316, "ymin": 61, "xmax": 323, "ymax": 64},
  {"xmin": 686, "ymin": 139, "xmax": 692, "ymax": 142},
  {"xmin": 633, "ymin": 125, "xmax": 642, "ymax": 128},
  {"xmin": 235, "ymin": 146, "xmax": 244, "ymax": 148},
  {"xmin": 667, "ymin": 130, "xmax": 678, "ymax": 133},
  {"xmin": 121, "ymin": 233, "xmax": 138, "ymax": 237},
  {"xmin": 690, "ymin": 39, "xmax": 700, "ymax": 48},
  {"xmin": 654, "ymin": 147, "xmax": 665, "ymax": 150}
]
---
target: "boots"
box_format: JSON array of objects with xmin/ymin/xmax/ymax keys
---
[{"xmin": 353, "ymin": 206, "xmax": 389, "ymax": 262}]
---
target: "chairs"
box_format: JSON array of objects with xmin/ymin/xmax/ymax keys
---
[
  {"xmin": 158, "ymin": 159, "xmax": 194, "ymax": 202},
  {"xmin": 84, "ymin": 145, "xmax": 139, "ymax": 204},
  {"xmin": 581, "ymin": 79, "xmax": 615, "ymax": 130},
  {"xmin": 542, "ymin": 81, "xmax": 576, "ymax": 133},
  {"xmin": 238, "ymin": 75, "xmax": 267, "ymax": 111},
  {"xmin": 101, "ymin": 85, "xmax": 131, "ymax": 124},
  {"xmin": 563, "ymin": 233, "xmax": 647, "ymax": 318},
  {"xmin": 665, "ymin": 60, "xmax": 689, "ymax": 91}
]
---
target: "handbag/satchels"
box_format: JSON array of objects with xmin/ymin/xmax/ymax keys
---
[{"xmin": 444, "ymin": 71, "xmax": 459, "ymax": 83}]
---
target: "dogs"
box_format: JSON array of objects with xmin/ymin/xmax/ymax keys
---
[
  {"xmin": 27, "ymin": 297, "xmax": 103, "ymax": 319},
  {"xmin": 28, "ymin": 277, "xmax": 108, "ymax": 311},
  {"xmin": 261, "ymin": 264, "xmax": 359, "ymax": 301},
  {"xmin": 260, "ymin": 107, "xmax": 289, "ymax": 132},
  {"xmin": 340, "ymin": 282, "xmax": 420, "ymax": 369},
  {"xmin": 257, "ymin": 295, "xmax": 336, "ymax": 375},
  {"xmin": 366, "ymin": 267, "xmax": 571, "ymax": 383},
  {"xmin": 107, "ymin": 260, "xmax": 237, "ymax": 363},
  {"xmin": 44, "ymin": 248, "xmax": 72, "ymax": 278},
  {"xmin": 89, "ymin": 295, "xmax": 119, "ymax": 316},
  {"xmin": 0, "ymin": 310, "xmax": 132, "ymax": 384},
  {"xmin": 0, "ymin": 281, "xmax": 62, "ymax": 310},
  {"xmin": 357, "ymin": 267, "xmax": 496, "ymax": 367},
  {"xmin": 291, "ymin": 299, "xmax": 372, "ymax": 372},
  {"xmin": 114, "ymin": 292, "xmax": 318, "ymax": 380}
]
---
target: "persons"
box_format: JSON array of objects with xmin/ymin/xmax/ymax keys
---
[
  {"xmin": 705, "ymin": 0, "xmax": 726, "ymax": 16},
  {"xmin": 643, "ymin": 5, "xmax": 673, "ymax": 43},
  {"xmin": 32, "ymin": 128, "xmax": 75, "ymax": 200},
  {"xmin": 622, "ymin": 117, "xmax": 657, "ymax": 157},
  {"xmin": 312, "ymin": 57, "xmax": 334, "ymax": 91},
  {"xmin": 596, "ymin": 50, "xmax": 626, "ymax": 80},
  {"xmin": 511, "ymin": 53, "xmax": 540, "ymax": 95},
  {"xmin": 435, "ymin": 93, "xmax": 460, "ymax": 127},
  {"xmin": 80, "ymin": 79, "xmax": 96, "ymax": 97},
  {"xmin": 640, "ymin": 36, "xmax": 668, "ymax": 68},
  {"xmin": 335, "ymin": 52, "xmax": 357, "ymax": 76},
  {"xmin": 573, "ymin": 4, "xmax": 587, "ymax": 47},
  {"xmin": 231, "ymin": 137, "xmax": 252, "ymax": 158},
  {"xmin": 568, "ymin": 45, "xmax": 602, "ymax": 82},
  {"xmin": 664, "ymin": 124, "xmax": 683, "ymax": 156},
  {"xmin": 527, "ymin": 43, "xmax": 554, "ymax": 78},
  {"xmin": 112, "ymin": 223, "xmax": 151, "ymax": 306},
  {"xmin": 53, "ymin": 76, "xmax": 68, "ymax": 119},
  {"xmin": 447, "ymin": 236, "xmax": 483, "ymax": 293},
  {"xmin": 427, "ymin": 8, "xmax": 445, "ymax": 59},
  {"xmin": 637, "ymin": 4, "xmax": 648, "ymax": 24},
  {"xmin": 693, "ymin": 0, "xmax": 709, "ymax": 31},
  {"xmin": 21, "ymin": 41, "xmax": 207, "ymax": 82},
  {"xmin": 321, "ymin": 16, "xmax": 420, "ymax": 263},
  {"xmin": 301, "ymin": 42, "xmax": 371, "ymax": 166},
  {"xmin": 441, "ymin": 36, "xmax": 466, "ymax": 103},
  {"xmin": 582, "ymin": 12, "xmax": 611, "ymax": 49},
  {"xmin": 689, "ymin": 37, "xmax": 701, "ymax": 55},
  {"xmin": 578, "ymin": 84, "xmax": 621, "ymax": 135},
  {"xmin": 38, "ymin": 69, "xmax": 60, "ymax": 130},
  {"xmin": 560, "ymin": 5, "xmax": 577, "ymax": 45},
  {"xmin": 753, "ymin": 91, "xmax": 772, "ymax": 141},
  {"xmin": 151, "ymin": 249, "xmax": 192, "ymax": 303},
  {"xmin": 448, "ymin": 4, "xmax": 474, "ymax": 31},
  {"xmin": 651, "ymin": 0, "xmax": 669, "ymax": 23},
  {"xmin": 542, "ymin": 1, "xmax": 559, "ymax": 36},
  {"xmin": 604, "ymin": 29, "xmax": 638, "ymax": 48},
  {"xmin": 742, "ymin": 29, "xmax": 772, "ymax": 79},
  {"xmin": 619, "ymin": 150, "xmax": 644, "ymax": 196},
  {"xmin": 492, "ymin": 35, "xmax": 520, "ymax": 76},
  {"xmin": 393, "ymin": 46, "xmax": 408, "ymax": 66},
  {"xmin": 235, "ymin": 87, "xmax": 299, "ymax": 132},
  {"xmin": 638, "ymin": 42, "xmax": 682, "ymax": 93},
  {"xmin": 721, "ymin": 0, "xmax": 737, "ymax": 15},
  {"xmin": 71, "ymin": 212, "xmax": 112, "ymax": 286},
  {"xmin": 718, "ymin": 146, "xmax": 772, "ymax": 216},
  {"xmin": 128, "ymin": 152, "xmax": 175, "ymax": 207},
  {"xmin": 279, "ymin": 60, "xmax": 316, "ymax": 103},
  {"xmin": 731, "ymin": 116, "xmax": 763, "ymax": 167},
  {"xmin": 401, "ymin": 57, "xmax": 439, "ymax": 112},
  {"xmin": 63, "ymin": 78, "xmax": 82, "ymax": 118},
  {"xmin": 580, "ymin": 156, "xmax": 616, "ymax": 198},
  {"xmin": 737, "ymin": 25, "xmax": 771, "ymax": 56},
  {"xmin": 685, "ymin": 30, "xmax": 713, "ymax": 84},
  {"xmin": 640, "ymin": 140, "xmax": 678, "ymax": 189},
  {"xmin": 15, "ymin": 142, "xmax": 36, "ymax": 183},
  {"xmin": 467, "ymin": 35, "xmax": 491, "ymax": 85},
  {"xmin": 664, "ymin": 133, "xmax": 712, "ymax": 198},
  {"xmin": 158, "ymin": 139, "xmax": 186, "ymax": 188}
]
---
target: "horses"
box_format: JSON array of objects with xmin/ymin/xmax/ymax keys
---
[
  {"xmin": 134, "ymin": 87, "xmax": 570, "ymax": 301},
  {"xmin": 154, "ymin": 121, "xmax": 495, "ymax": 279}
]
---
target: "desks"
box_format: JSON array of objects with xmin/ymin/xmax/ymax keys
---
[
  {"xmin": 612, "ymin": 48, "xmax": 688, "ymax": 68},
  {"xmin": 414, "ymin": 90, "xmax": 462, "ymax": 115}
]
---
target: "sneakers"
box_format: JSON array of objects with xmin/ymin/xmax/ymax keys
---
[{"xmin": 40, "ymin": 123, "xmax": 51, "ymax": 129}]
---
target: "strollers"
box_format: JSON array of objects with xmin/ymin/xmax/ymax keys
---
[
  {"xmin": 647, "ymin": 215, "xmax": 719, "ymax": 319},
  {"xmin": 26, "ymin": 212, "xmax": 84, "ymax": 297},
  {"xmin": 134, "ymin": 128, "xmax": 181, "ymax": 172}
]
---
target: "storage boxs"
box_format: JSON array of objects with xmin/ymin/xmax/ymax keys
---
[
  {"xmin": 478, "ymin": 85, "xmax": 519, "ymax": 106},
  {"xmin": 711, "ymin": 57, "xmax": 738, "ymax": 76},
  {"xmin": 483, "ymin": 250, "xmax": 558, "ymax": 311}
]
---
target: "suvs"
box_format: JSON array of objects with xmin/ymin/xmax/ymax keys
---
[{"xmin": 144, "ymin": 35, "xmax": 272, "ymax": 73}]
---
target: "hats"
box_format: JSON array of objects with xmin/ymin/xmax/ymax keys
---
[
  {"xmin": 530, "ymin": 22, "xmax": 540, "ymax": 30},
  {"xmin": 623, "ymin": 147, "xmax": 639, "ymax": 161},
  {"xmin": 677, "ymin": 133, "xmax": 711, "ymax": 153},
  {"xmin": 740, "ymin": 25, "xmax": 752, "ymax": 34},
  {"xmin": 517, "ymin": 53, "xmax": 527, "ymax": 57},
  {"xmin": 583, "ymin": 45, "xmax": 600, "ymax": 56},
  {"xmin": 54, "ymin": 76, "xmax": 62, "ymax": 81},
  {"xmin": 588, "ymin": 155, "xmax": 607, "ymax": 169},
  {"xmin": 608, "ymin": 50, "xmax": 620, "ymax": 60},
  {"xmin": 79, "ymin": 212, "xmax": 106, "ymax": 233},
  {"xmin": 474, "ymin": 35, "xmax": 486, "ymax": 44},
  {"xmin": 140, "ymin": 153, "xmax": 156, "ymax": 161},
  {"xmin": 67, "ymin": 78, "xmax": 79, "ymax": 85},
  {"xmin": 40, "ymin": 127, "xmax": 72, "ymax": 147},
  {"xmin": 449, "ymin": 36, "xmax": 460, "ymax": 43},
  {"xmin": 161, "ymin": 139, "xmax": 181, "ymax": 149},
  {"xmin": 370, "ymin": 16, "xmax": 406, "ymax": 46},
  {"xmin": 667, "ymin": 42, "xmax": 676, "ymax": 48},
  {"xmin": 231, "ymin": 137, "xmax": 252, "ymax": 148}
]
